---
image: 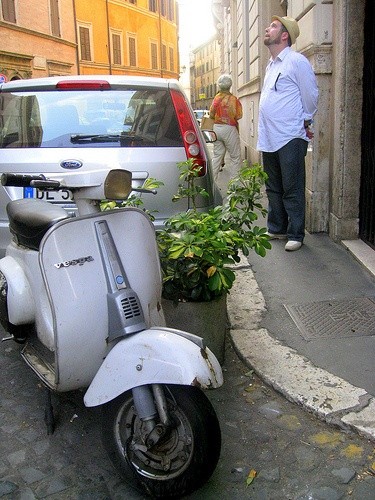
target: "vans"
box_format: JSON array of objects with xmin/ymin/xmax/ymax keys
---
[{"xmin": 0, "ymin": 74, "xmax": 218, "ymax": 267}]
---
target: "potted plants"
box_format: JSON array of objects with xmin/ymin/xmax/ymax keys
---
[{"xmin": 94, "ymin": 158, "xmax": 273, "ymax": 364}]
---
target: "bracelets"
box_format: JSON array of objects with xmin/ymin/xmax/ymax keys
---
[{"xmin": 305, "ymin": 123, "xmax": 311, "ymax": 129}]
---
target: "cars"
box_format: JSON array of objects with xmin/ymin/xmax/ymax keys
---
[{"xmin": 192, "ymin": 110, "xmax": 211, "ymax": 126}]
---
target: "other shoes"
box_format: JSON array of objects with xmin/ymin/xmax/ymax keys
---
[
  {"xmin": 285, "ymin": 241, "xmax": 303, "ymax": 251},
  {"xmin": 256, "ymin": 232, "xmax": 287, "ymax": 240}
]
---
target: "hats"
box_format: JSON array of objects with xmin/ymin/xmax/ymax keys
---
[{"xmin": 271, "ymin": 15, "xmax": 300, "ymax": 44}]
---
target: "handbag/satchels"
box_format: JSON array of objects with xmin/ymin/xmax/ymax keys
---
[{"xmin": 200, "ymin": 117, "xmax": 214, "ymax": 131}]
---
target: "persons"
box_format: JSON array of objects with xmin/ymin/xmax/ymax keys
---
[
  {"xmin": 205, "ymin": 75, "xmax": 243, "ymax": 190},
  {"xmin": 256, "ymin": 14, "xmax": 319, "ymax": 251}
]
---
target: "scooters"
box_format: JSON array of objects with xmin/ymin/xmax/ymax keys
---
[{"xmin": 0, "ymin": 168, "xmax": 224, "ymax": 500}]
